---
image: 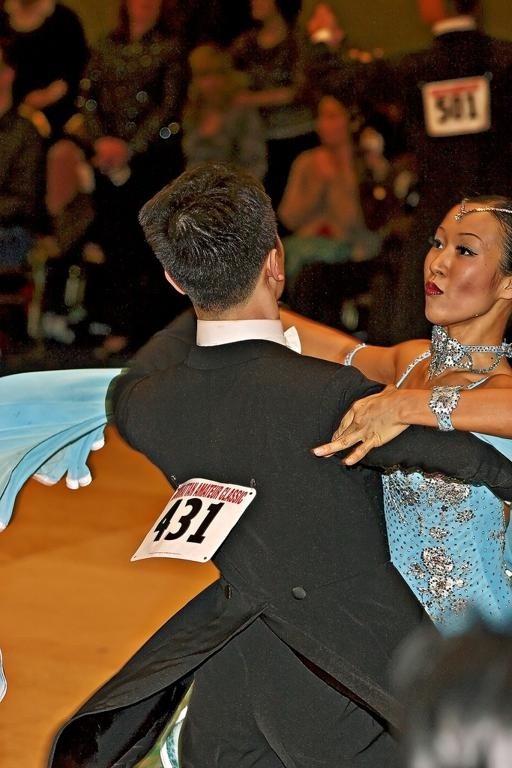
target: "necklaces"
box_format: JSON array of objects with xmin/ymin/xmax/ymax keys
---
[{"xmin": 426, "ymin": 325, "xmax": 512, "ymax": 379}]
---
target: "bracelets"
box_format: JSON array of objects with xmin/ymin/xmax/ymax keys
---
[{"xmin": 429, "ymin": 384, "xmax": 459, "ymax": 432}]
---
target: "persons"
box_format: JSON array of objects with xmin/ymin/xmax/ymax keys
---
[
  {"xmin": 279, "ymin": 195, "xmax": 512, "ymax": 768},
  {"xmin": 2, "ymin": 0, "xmax": 511, "ymax": 358},
  {"xmin": 47, "ymin": 164, "xmax": 512, "ymax": 768}
]
